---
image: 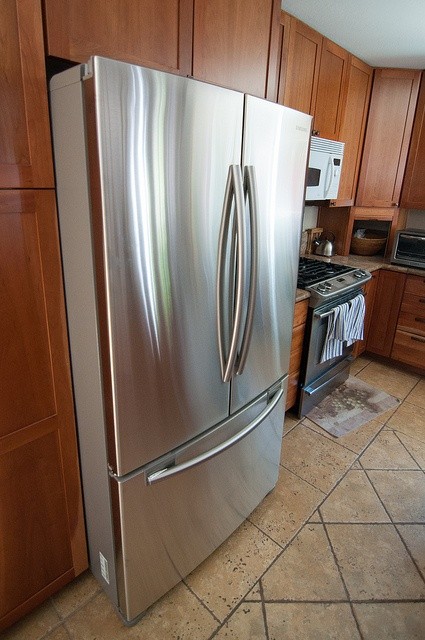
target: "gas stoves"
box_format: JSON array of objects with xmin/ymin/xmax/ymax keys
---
[{"xmin": 297, "ymin": 257, "xmax": 372, "ymax": 309}]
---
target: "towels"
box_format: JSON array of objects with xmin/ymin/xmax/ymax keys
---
[
  {"xmin": 320, "ymin": 303, "xmax": 350, "ymax": 364},
  {"xmin": 346, "ymin": 294, "xmax": 366, "ymax": 349}
]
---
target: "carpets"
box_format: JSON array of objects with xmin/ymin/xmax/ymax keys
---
[{"xmin": 304, "ymin": 373, "xmax": 402, "ymax": 439}]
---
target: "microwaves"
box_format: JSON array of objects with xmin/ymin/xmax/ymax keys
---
[{"xmin": 304, "ymin": 135, "xmax": 346, "ymax": 201}]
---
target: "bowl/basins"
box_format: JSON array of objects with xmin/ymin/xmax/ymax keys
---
[{"xmin": 351, "ymin": 228, "xmax": 386, "ymax": 255}]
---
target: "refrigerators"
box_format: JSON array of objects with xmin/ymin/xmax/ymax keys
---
[{"xmin": 48, "ymin": 55, "xmax": 315, "ymax": 629}]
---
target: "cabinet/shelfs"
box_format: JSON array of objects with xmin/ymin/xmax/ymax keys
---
[
  {"xmin": 277, "ymin": 9, "xmax": 292, "ymax": 106},
  {"xmin": 1, "ymin": 1, "xmax": 57, "ymax": 186},
  {"xmin": 316, "ymin": 205, "xmax": 409, "ymax": 258},
  {"xmin": 1, "ymin": 188, "xmax": 108, "ymax": 636},
  {"xmin": 284, "ymin": 298, "xmax": 312, "ymax": 413},
  {"xmin": 352, "ymin": 272, "xmax": 379, "ymax": 361},
  {"xmin": 283, "ymin": 15, "xmax": 350, "ymax": 141},
  {"xmin": 328, "ymin": 54, "xmax": 374, "ymax": 208},
  {"xmin": 367, "ymin": 270, "xmax": 407, "ymax": 360},
  {"xmin": 193, "ymin": 1, "xmax": 283, "ymax": 102},
  {"xmin": 355, "ymin": 67, "xmax": 423, "ymax": 208},
  {"xmin": 42, "ymin": 0, "xmax": 179, "ymax": 78},
  {"xmin": 390, "ymin": 274, "xmax": 425, "ymax": 377},
  {"xmin": 399, "ymin": 68, "xmax": 425, "ymax": 210}
]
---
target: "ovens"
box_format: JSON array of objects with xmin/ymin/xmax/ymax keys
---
[
  {"xmin": 299, "ymin": 287, "xmax": 372, "ymax": 423},
  {"xmin": 389, "ymin": 226, "xmax": 425, "ymax": 270}
]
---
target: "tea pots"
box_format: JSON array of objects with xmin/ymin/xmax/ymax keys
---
[{"xmin": 314, "ymin": 231, "xmax": 336, "ymax": 257}]
